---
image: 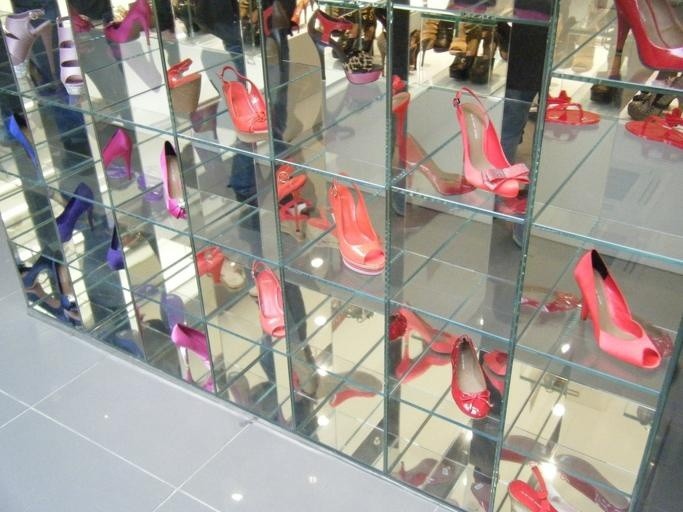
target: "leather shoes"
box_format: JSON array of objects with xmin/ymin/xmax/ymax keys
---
[
  {"xmin": 558, "ymin": 454, "xmax": 630, "ymax": 511},
  {"xmin": 449, "ymin": 29, "xmax": 482, "ymax": 79},
  {"xmin": 455, "ymin": 87, "xmax": 530, "ymax": 199},
  {"xmin": 468, "ymin": 28, "xmax": 498, "ymax": 84},
  {"xmin": 106, "ymin": 224, "xmax": 124, "ymax": 270},
  {"xmin": 315, "ymin": 371, "xmax": 382, "ymax": 406},
  {"xmin": 451, "ymin": 336, "xmax": 492, "ymax": 419},
  {"xmin": 160, "ymin": 141, "xmax": 187, "ymax": 220},
  {"xmin": 7, "ymin": 111, "xmax": 38, "ymax": 166}
]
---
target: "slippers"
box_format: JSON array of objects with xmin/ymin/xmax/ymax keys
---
[{"xmin": 225, "ymin": 259, "xmax": 245, "ymax": 291}]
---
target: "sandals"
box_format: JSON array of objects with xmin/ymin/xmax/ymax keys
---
[
  {"xmin": 625, "ymin": 109, "xmax": 683, "ymax": 148},
  {"xmin": 308, "ymin": 207, "xmax": 338, "ymax": 244},
  {"xmin": 221, "ymin": 66, "xmax": 269, "ymax": 143},
  {"xmin": 250, "ymin": 261, "xmax": 285, "ymax": 338},
  {"xmin": 328, "ymin": 171, "xmax": 386, "ymax": 276},
  {"xmin": 168, "ymin": 59, "xmax": 203, "ymax": 115},
  {"xmin": 546, "ymin": 90, "xmax": 599, "ymax": 124},
  {"xmin": 279, "ymin": 199, "xmax": 311, "ymax": 241},
  {"xmin": 57, "ymin": 16, "xmax": 87, "ymax": 97}
]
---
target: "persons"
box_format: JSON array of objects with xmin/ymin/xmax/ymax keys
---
[
  {"xmin": 489, "ymin": 0, "xmax": 558, "ymax": 248},
  {"xmin": 190, "ymin": 1, "xmax": 295, "ymax": 232}
]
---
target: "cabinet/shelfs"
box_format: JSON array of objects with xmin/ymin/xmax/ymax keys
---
[{"xmin": 1, "ymin": 0, "xmax": 682, "ymax": 512}]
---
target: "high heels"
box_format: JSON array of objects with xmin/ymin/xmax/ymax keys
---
[
  {"xmin": 409, "ymin": 29, "xmax": 422, "ymax": 71},
  {"xmin": 507, "ymin": 465, "xmax": 558, "ymax": 512},
  {"xmin": 421, "ymin": 20, "xmax": 439, "ymax": 67},
  {"xmin": 104, "ymin": 1, "xmax": 153, "ymax": 45},
  {"xmin": 5, "ymin": 9, "xmax": 57, "ymax": 75},
  {"xmin": 617, "ymin": 0, "xmax": 683, "ymax": 71},
  {"xmin": 111, "ymin": 47, "xmax": 160, "ymax": 92},
  {"xmin": 392, "ymin": 74, "xmax": 411, "ymax": 146},
  {"xmin": 399, "ymin": 457, "xmax": 439, "ymax": 487},
  {"xmin": 18, "ymin": 248, "xmax": 63, "ymax": 293},
  {"xmin": 308, "ymin": 9, "xmax": 383, "ymax": 85},
  {"xmin": 575, "ymin": 250, "xmax": 662, "ymax": 370},
  {"xmin": 53, "ymin": 253, "xmax": 79, "ymax": 312},
  {"xmin": 54, "ymin": 182, "xmax": 95, "ymax": 243},
  {"xmin": 265, "ymin": 1, "xmax": 298, "ymax": 67},
  {"xmin": 400, "ymin": 306, "xmax": 461, "ymax": 354},
  {"xmin": 629, "ymin": 70, "xmax": 669, "ymax": 115},
  {"xmin": 104, "ymin": 127, "xmax": 132, "ymax": 181},
  {"xmin": 396, "ymin": 346, "xmax": 451, "ymax": 382},
  {"xmin": 183, "ymin": 366, "xmax": 213, "ymax": 391},
  {"xmin": 199, "ymin": 246, "xmax": 223, "ymax": 282},
  {"xmin": 276, "ymin": 165, "xmax": 306, "ymax": 233},
  {"xmin": 650, "ymin": 76, "xmax": 683, "ymax": 112},
  {"xmin": 399, "ymin": 131, "xmax": 476, "ymax": 195},
  {"xmin": 171, "ymin": 322, "xmax": 208, "ymax": 366}
]
